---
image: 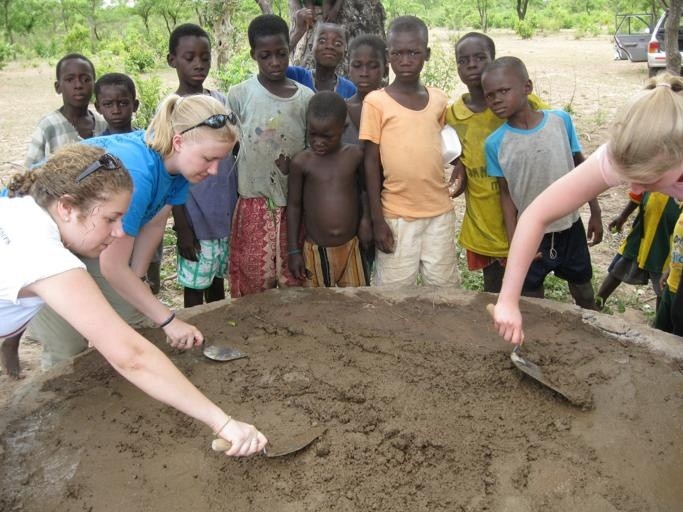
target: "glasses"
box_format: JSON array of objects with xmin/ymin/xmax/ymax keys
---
[
  {"xmin": 75, "ymin": 153, "xmax": 121, "ymax": 182},
  {"xmin": 180, "ymin": 111, "xmax": 237, "ymax": 135}
]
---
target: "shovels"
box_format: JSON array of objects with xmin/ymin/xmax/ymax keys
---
[
  {"xmin": 163, "ymin": 335, "xmax": 248, "ymax": 362},
  {"xmin": 485, "ymin": 303, "xmax": 572, "ymax": 400},
  {"xmin": 211, "ymin": 426, "xmax": 329, "ymax": 458}
]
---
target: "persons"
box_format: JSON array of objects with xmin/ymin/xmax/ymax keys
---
[
  {"xmin": 166, "ymin": 23, "xmax": 239, "ymax": 309},
  {"xmin": 23, "ymin": 53, "xmax": 109, "ymax": 168},
  {"xmin": 93, "ymin": 72, "xmax": 164, "ymax": 296},
  {"xmin": 445, "ymin": 31, "xmax": 552, "ymax": 295},
  {"xmin": 0, "ymin": 92, "xmax": 241, "ymax": 382},
  {"xmin": 480, "ymin": 55, "xmax": 604, "ymax": 312},
  {"xmin": 227, "ymin": 13, "xmax": 315, "ymax": 297},
  {"xmin": 0, "ymin": 143, "xmax": 269, "ymax": 457},
  {"xmin": 340, "ymin": 33, "xmax": 390, "ymax": 145},
  {"xmin": 492, "ymin": 71, "xmax": 683, "ymax": 347},
  {"xmin": 285, "ymin": 89, "xmax": 374, "ymax": 288},
  {"xmin": 285, "ymin": 7, "xmax": 358, "ymax": 100},
  {"xmin": 358, "ymin": 14, "xmax": 461, "ymax": 289}
]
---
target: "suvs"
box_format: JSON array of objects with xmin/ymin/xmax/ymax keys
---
[{"xmin": 612, "ymin": 10, "xmax": 683, "ymax": 78}]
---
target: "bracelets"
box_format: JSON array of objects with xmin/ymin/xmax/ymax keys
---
[
  {"xmin": 287, "ymin": 249, "xmax": 301, "ymax": 255},
  {"xmin": 160, "ymin": 313, "xmax": 176, "ymax": 328}
]
